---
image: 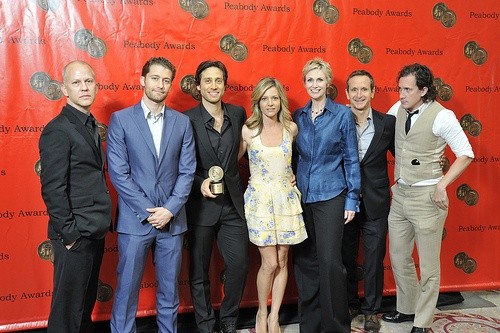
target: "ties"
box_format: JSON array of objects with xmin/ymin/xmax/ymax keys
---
[{"xmin": 405, "ymin": 110, "xmax": 419, "ymax": 136}]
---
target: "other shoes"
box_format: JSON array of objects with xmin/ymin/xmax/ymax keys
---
[
  {"xmin": 255, "ymin": 312, "xmax": 267, "ymax": 333},
  {"xmin": 267, "ymin": 313, "xmax": 281, "ymax": 333}
]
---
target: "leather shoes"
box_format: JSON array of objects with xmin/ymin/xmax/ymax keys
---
[
  {"xmin": 411, "ymin": 326, "xmax": 426, "ymax": 333},
  {"xmin": 381, "ymin": 310, "xmax": 415, "ymax": 323}
]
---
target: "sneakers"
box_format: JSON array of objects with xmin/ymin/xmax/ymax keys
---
[
  {"xmin": 222, "ymin": 323, "xmax": 237, "ymax": 333},
  {"xmin": 365, "ymin": 314, "xmax": 380, "ymax": 332},
  {"xmin": 349, "ymin": 309, "xmax": 358, "ymax": 317}
]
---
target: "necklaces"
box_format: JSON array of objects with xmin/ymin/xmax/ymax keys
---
[{"xmin": 312, "ymin": 106, "xmax": 324, "ymax": 113}]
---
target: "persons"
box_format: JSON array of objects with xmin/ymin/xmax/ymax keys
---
[
  {"xmin": 345, "ymin": 61, "xmax": 475, "ymax": 333},
  {"xmin": 292, "ymin": 56, "xmax": 362, "ymax": 332},
  {"xmin": 38, "ymin": 60, "xmax": 112, "ymax": 333},
  {"xmin": 106, "ymin": 56, "xmax": 197, "ymax": 332},
  {"xmin": 341, "ymin": 68, "xmax": 446, "ymax": 333},
  {"xmin": 179, "ymin": 58, "xmax": 296, "ymax": 333},
  {"xmin": 237, "ymin": 77, "xmax": 308, "ymax": 332}
]
